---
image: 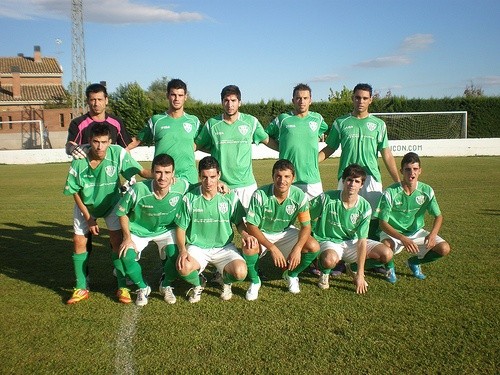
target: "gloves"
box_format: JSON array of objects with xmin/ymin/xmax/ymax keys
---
[
  {"xmin": 65, "ymin": 141, "xmax": 89, "ymax": 160},
  {"xmin": 120, "ymin": 175, "xmax": 136, "ymax": 196}
]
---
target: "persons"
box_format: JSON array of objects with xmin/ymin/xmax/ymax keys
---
[
  {"xmin": 309, "ymin": 164, "xmax": 393, "ymax": 294},
  {"xmin": 264, "ymin": 85, "xmax": 331, "ymax": 206},
  {"xmin": 318, "ymin": 83, "xmax": 401, "ymax": 275},
  {"xmin": 193, "ymin": 84, "xmax": 279, "ymax": 213},
  {"xmin": 63, "ymin": 122, "xmax": 178, "ymax": 304},
  {"xmin": 372, "ymin": 152, "xmax": 451, "ymax": 284},
  {"xmin": 114, "ymin": 153, "xmax": 258, "ymax": 307},
  {"xmin": 65, "ymin": 83, "xmax": 136, "ymax": 185},
  {"xmin": 241, "ymin": 159, "xmax": 321, "ymax": 301},
  {"xmin": 126, "ymin": 79, "xmax": 210, "ymax": 185}
]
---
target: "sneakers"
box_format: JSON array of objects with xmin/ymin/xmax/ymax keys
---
[
  {"xmin": 282, "ymin": 269, "xmax": 299, "ymax": 293},
  {"xmin": 318, "ymin": 271, "xmax": 329, "ymax": 289},
  {"xmin": 385, "ymin": 268, "xmax": 396, "ymax": 283},
  {"xmin": 408, "ymin": 258, "xmax": 427, "ymax": 280},
  {"xmin": 67, "ymin": 287, "xmax": 89, "ymax": 304},
  {"xmin": 116, "ymin": 288, "xmax": 132, "ymax": 304},
  {"xmin": 186, "ymin": 284, "xmax": 204, "ymax": 303},
  {"xmin": 346, "ymin": 263, "xmax": 368, "ymax": 286},
  {"xmin": 135, "ymin": 286, "xmax": 151, "ymax": 306},
  {"xmin": 159, "ymin": 281, "xmax": 177, "ymax": 304},
  {"xmin": 245, "ymin": 276, "xmax": 261, "ymax": 301},
  {"xmin": 220, "ymin": 279, "xmax": 232, "ymax": 300}
]
